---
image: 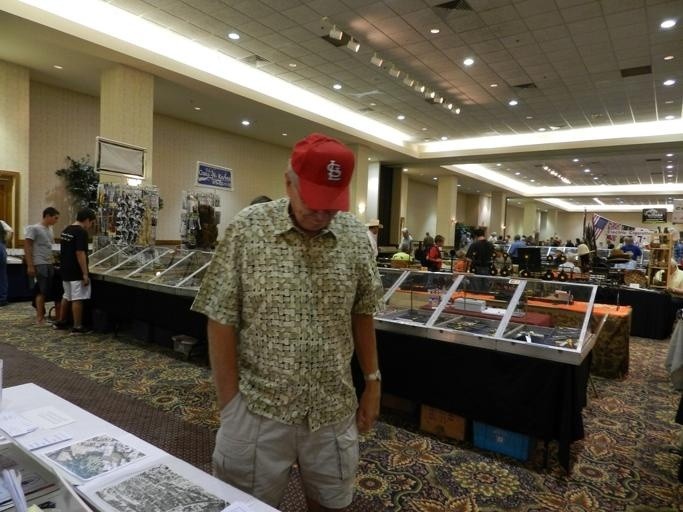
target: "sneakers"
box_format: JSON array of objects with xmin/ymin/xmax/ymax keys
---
[
  {"xmin": 72, "ymin": 324, "xmax": 95, "ymax": 334},
  {"xmin": 52, "ymin": 319, "xmax": 70, "ymax": 329}
]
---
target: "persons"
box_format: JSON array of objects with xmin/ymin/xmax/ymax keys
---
[
  {"xmin": 191, "ymin": 134, "xmax": 384, "ymax": 511},
  {"xmin": 398, "ymin": 227, "xmax": 413, "ymax": 255},
  {"xmin": 0, "ymin": 220, "xmax": 14, "ymax": 307},
  {"xmin": 422, "ymin": 232, "xmax": 445, "ymax": 294},
  {"xmin": 464, "ymin": 227, "xmax": 495, "ymax": 295},
  {"xmin": 51, "ymin": 207, "xmax": 96, "ymax": 336},
  {"xmin": 22, "ymin": 207, "xmax": 59, "ymax": 326},
  {"xmin": 365, "ymin": 226, "xmax": 379, "ymax": 261},
  {"xmin": 488, "ymin": 232, "xmax": 683, "ymax": 294}
]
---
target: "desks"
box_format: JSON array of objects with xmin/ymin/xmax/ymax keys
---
[
  {"xmin": 560, "ymin": 281, "xmax": 676, "ymax": 340},
  {"xmin": 389, "ymin": 289, "xmax": 633, "ymax": 380}
]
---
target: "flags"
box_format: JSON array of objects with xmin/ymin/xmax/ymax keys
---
[
  {"xmin": 591, "ymin": 214, "xmax": 608, "ymax": 240},
  {"xmin": 606, "ymin": 221, "xmax": 621, "ymax": 242}
]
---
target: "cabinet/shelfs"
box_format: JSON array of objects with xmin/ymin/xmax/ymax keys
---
[
  {"xmin": 647, "ymin": 232, "xmax": 672, "ymax": 289},
  {"xmin": 84, "ymin": 243, "xmax": 609, "ymax": 470}
]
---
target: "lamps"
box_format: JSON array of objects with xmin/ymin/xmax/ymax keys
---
[{"xmin": 329, "ymin": 24, "xmax": 461, "ymax": 114}]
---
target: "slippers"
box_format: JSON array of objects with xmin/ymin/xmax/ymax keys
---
[{"xmin": 32, "ymin": 317, "xmax": 55, "ymax": 326}]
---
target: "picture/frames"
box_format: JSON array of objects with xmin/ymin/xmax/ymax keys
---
[{"xmin": 194, "ymin": 161, "xmax": 233, "ymax": 192}]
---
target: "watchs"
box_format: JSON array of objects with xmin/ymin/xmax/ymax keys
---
[{"xmin": 363, "ymin": 370, "xmax": 382, "ymax": 382}]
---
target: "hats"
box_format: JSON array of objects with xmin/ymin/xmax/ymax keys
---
[
  {"xmin": 364, "ymin": 219, "xmax": 383, "ymax": 229},
  {"xmin": 292, "ymin": 133, "xmax": 354, "ymax": 212}
]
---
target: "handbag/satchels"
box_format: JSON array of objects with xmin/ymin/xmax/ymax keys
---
[{"xmin": 421, "ymin": 255, "xmax": 437, "ymax": 269}]
